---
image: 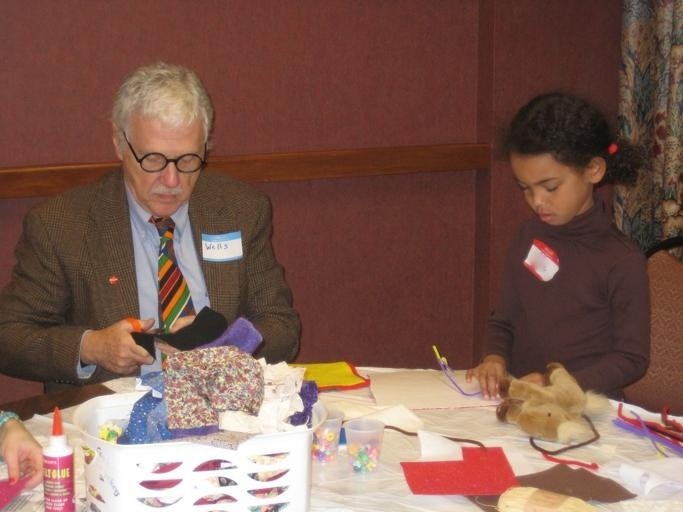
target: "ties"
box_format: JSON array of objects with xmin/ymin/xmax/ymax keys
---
[{"xmin": 149, "ymin": 217, "xmax": 198, "ymax": 372}]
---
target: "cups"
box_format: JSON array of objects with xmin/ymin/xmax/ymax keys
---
[
  {"xmin": 312, "ymin": 408, "xmax": 347, "ymax": 471},
  {"xmin": 340, "ymin": 417, "xmax": 385, "ymax": 478}
]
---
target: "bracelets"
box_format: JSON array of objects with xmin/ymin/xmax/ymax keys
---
[{"xmin": 0, "ymin": 411, "xmax": 22, "ymax": 428}]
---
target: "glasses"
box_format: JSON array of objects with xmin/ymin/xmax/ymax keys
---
[{"xmin": 123, "ymin": 130, "xmax": 208, "ymax": 174}]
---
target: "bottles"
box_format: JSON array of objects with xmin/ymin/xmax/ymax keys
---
[{"xmin": 41, "ymin": 405, "xmax": 76, "ymax": 512}]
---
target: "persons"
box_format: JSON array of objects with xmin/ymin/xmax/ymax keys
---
[
  {"xmin": 465, "ymin": 90, "xmax": 652, "ymax": 402},
  {"xmin": 0, "ymin": 59, "xmax": 302, "ymax": 392},
  {"xmin": 0, "ymin": 407, "xmax": 44, "ymax": 491}
]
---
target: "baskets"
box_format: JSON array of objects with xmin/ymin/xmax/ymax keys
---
[{"xmin": 74, "ymin": 392, "xmax": 327, "ymax": 512}]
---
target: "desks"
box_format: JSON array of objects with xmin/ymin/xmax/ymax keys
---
[{"xmin": 0, "ymin": 363, "xmax": 683, "ymax": 509}]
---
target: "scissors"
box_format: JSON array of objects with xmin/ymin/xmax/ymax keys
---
[{"xmin": 128, "ymin": 317, "xmax": 167, "ymax": 344}]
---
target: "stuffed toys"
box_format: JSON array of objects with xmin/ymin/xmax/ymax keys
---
[{"xmin": 492, "ymin": 361, "xmax": 612, "ymax": 443}]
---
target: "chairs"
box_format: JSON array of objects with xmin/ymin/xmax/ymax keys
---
[{"xmin": 624, "ymin": 235, "xmax": 683, "ymax": 411}]
